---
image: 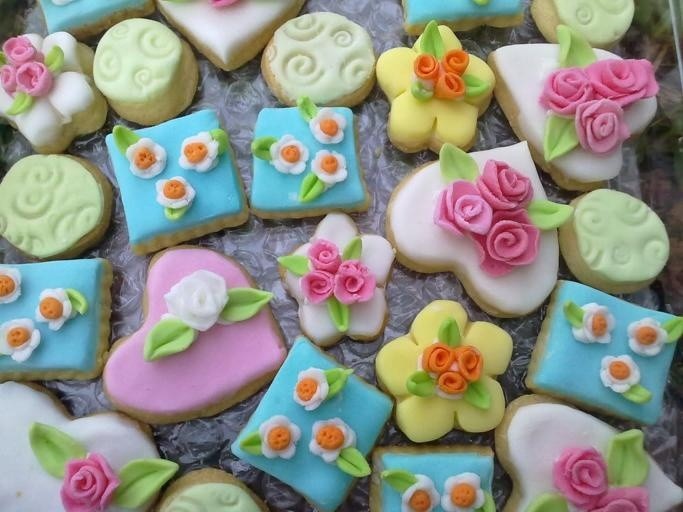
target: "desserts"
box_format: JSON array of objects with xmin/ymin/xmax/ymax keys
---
[{"xmin": 0, "ymin": 0, "xmax": 683, "ymax": 512}]
